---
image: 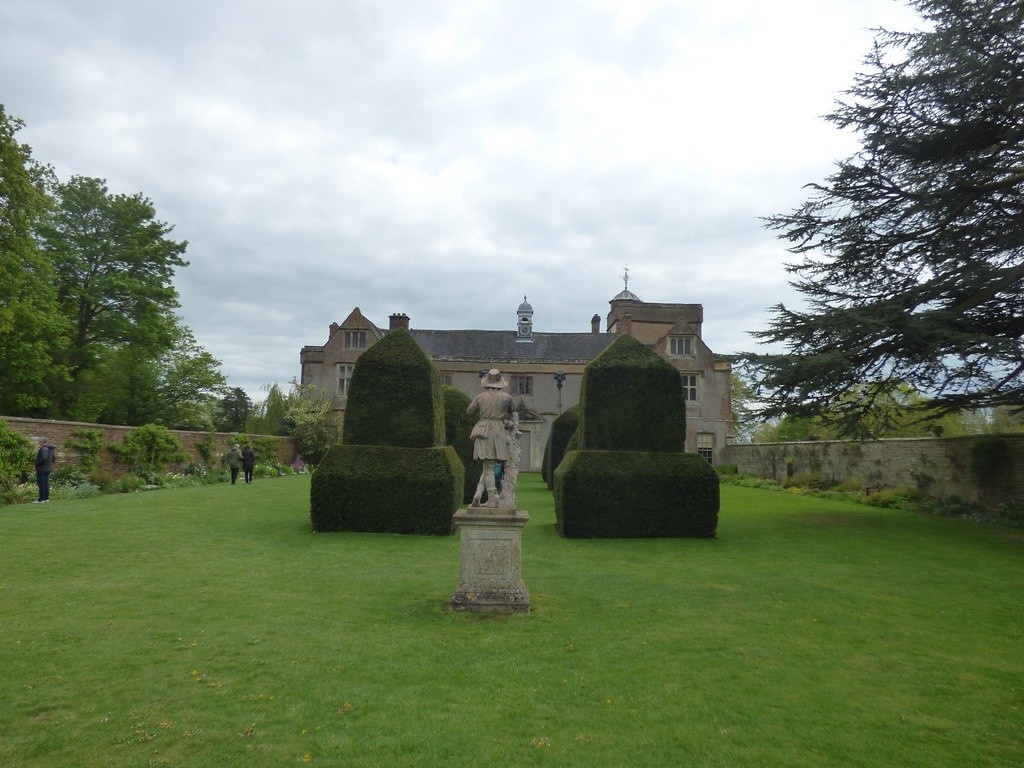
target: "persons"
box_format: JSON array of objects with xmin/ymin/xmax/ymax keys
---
[
  {"xmin": 32, "ymin": 437, "xmax": 55, "ymax": 504},
  {"xmin": 226, "ymin": 443, "xmax": 254, "ymax": 485},
  {"xmin": 467, "ymin": 368, "xmax": 517, "ymax": 507}
]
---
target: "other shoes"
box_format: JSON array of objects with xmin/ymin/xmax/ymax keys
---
[{"xmin": 248, "ymin": 481, "xmax": 252, "ymax": 485}]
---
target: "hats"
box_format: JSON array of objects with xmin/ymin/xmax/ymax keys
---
[{"xmin": 244, "ymin": 443, "xmax": 252, "ymax": 448}]
